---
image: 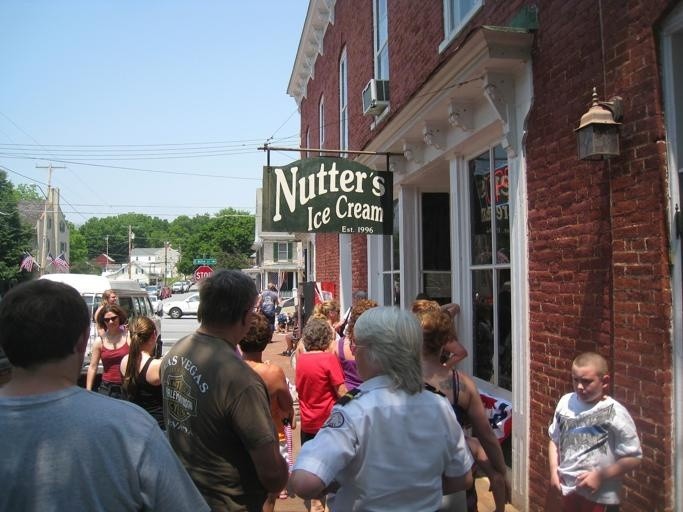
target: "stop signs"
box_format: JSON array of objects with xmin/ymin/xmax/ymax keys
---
[{"xmin": 194, "ymin": 266, "xmax": 214, "ymax": 283}]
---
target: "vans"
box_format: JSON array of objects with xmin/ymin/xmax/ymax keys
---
[{"xmin": 34, "ymin": 270, "xmax": 162, "ymax": 393}]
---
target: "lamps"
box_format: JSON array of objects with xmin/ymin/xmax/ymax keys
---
[{"xmin": 570, "ymin": 84, "xmax": 628, "ymax": 164}]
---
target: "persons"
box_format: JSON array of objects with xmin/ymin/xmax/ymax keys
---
[
  {"xmin": 1, "ymin": 269, "xmax": 511, "ymax": 512},
  {"xmin": 547, "ymin": 352, "xmax": 643, "ymax": 512}
]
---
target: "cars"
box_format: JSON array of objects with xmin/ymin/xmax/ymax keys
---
[
  {"xmin": 143, "ymin": 277, "xmax": 195, "ymax": 318},
  {"xmin": 160, "ymin": 294, "xmax": 200, "ymax": 320}
]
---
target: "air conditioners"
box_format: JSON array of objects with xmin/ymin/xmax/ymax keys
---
[{"xmin": 360, "ymin": 77, "xmax": 390, "ymax": 116}]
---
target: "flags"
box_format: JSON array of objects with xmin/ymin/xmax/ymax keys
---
[
  {"xmin": 17, "ymin": 253, "xmax": 34, "ymax": 273},
  {"xmin": 47, "ymin": 253, "xmax": 55, "ymax": 265},
  {"xmin": 53, "ymin": 254, "xmax": 71, "ymax": 272}
]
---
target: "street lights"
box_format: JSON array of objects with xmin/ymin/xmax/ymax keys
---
[{"xmin": 127, "ymin": 231, "xmax": 136, "ymax": 280}]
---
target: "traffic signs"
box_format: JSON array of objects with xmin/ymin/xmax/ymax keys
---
[{"xmin": 193, "ymin": 258, "xmax": 216, "ymax": 265}]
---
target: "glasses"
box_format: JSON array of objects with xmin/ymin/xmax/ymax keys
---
[{"xmin": 103, "ymin": 316, "xmax": 118, "ymax": 322}]
